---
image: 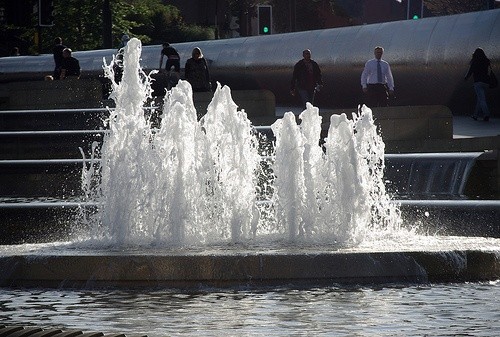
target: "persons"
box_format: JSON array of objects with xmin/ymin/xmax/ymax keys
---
[
  {"xmin": 118, "ymin": 33, "xmax": 130, "ymax": 49},
  {"xmin": 11, "ymin": 47, "xmax": 21, "ymax": 56},
  {"xmin": 288, "ymin": 48, "xmax": 321, "ymax": 108},
  {"xmin": 160, "ymin": 42, "xmax": 180, "ymax": 72},
  {"xmin": 185, "ymin": 46, "xmax": 211, "ymax": 92},
  {"xmin": 465, "ymin": 48, "xmax": 492, "ymax": 121},
  {"xmin": 361, "ymin": 46, "xmax": 394, "ymax": 106},
  {"xmin": 53, "ymin": 36, "xmax": 80, "ymax": 80}
]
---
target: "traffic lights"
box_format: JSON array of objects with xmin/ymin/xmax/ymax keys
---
[
  {"xmin": 257, "ymin": 5, "xmax": 272, "ymax": 35},
  {"xmin": 406, "ymin": 0, "xmax": 424, "ymax": 21},
  {"xmin": 39, "ymin": 0, "xmax": 56, "ymax": 27},
  {"xmin": 488, "ymin": 0, "xmax": 496, "ymax": 9}
]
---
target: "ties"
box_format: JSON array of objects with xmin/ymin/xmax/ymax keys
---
[{"xmin": 377, "ymin": 60, "xmax": 383, "ymax": 82}]
